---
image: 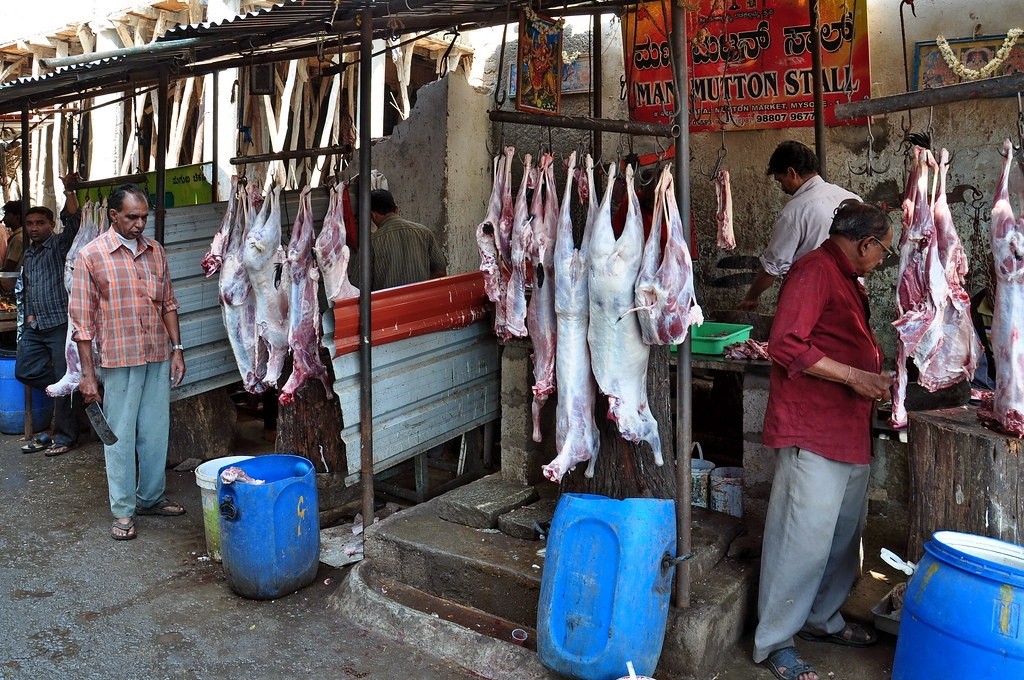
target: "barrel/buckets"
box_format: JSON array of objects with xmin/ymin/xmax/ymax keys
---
[
  {"xmin": 194, "ymin": 456, "xmax": 256, "ymax": 562},
  {"xmin": 709, "ymin": 466, "xmax": 743, "ymax": 519},
  {"xmin": 217, "ymin": 454, "xmax": 320, "ymax": 600},
  {"xmin": 675, "ymin": 458, "xmax": 715, "ymax": 508},
  {"xmin": 536, "ymin": 493, "xmax": 678, "ymax": 680},
  {"xmin": 891, "ymin": 530, "xmax": 1024, "ymax": 680},
  {"xmin": 0, "ymin": 340, "xmax": 54, "ymax": 435}
]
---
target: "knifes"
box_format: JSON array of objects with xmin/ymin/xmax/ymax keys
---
[
  {"xmin": 85, "ymin": 400, "xmax": 119, "ymax": 445},
  {"xmin": 715, "ymin": 256, "xmax": 762, "ymax": 269}
]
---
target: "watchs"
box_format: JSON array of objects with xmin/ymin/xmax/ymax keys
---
[{"xmin": 173, "ymin": 344, "xmax": 184, "ymax": 351}]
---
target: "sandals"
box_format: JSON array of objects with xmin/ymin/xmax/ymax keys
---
[
  {"xmin": 763, "ymin": 647, "xmax": 818, "ymax": 680},
  {"xmin": 799, "ymin": 620, "xmax": 875, "ymax": 646},
  {"xmin": 111, "ymin": 516, "xmax": 136, "ymax": 540},
  {"xmin": 133, "ymin": 498, "xmax": 184, "ymax": 516}
]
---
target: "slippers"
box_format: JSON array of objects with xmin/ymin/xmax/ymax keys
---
[
  {"xmin": 44, "ymin": 444, "xmax": 68, "ymax": 456},
  {"xmin": 20, "ymin": 433, "xmax": 55, "ymax": 452}
]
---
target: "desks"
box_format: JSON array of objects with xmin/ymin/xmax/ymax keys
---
[{"xmin": 911, "ymin": 34, "xmax": 1024, "ymax": 98}]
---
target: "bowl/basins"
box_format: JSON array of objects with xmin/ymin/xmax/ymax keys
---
[{"xmin": 0, "ymin": 272, "xmax": 20, "ymax": 292}]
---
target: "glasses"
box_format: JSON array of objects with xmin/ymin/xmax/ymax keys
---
[{"xmin": 863, "ymin": 236, "xmax": 893, "ymax": 260}]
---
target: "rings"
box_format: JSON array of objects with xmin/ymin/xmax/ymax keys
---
[{"xmin": 877, "ymin": 398, "xmax": 882, "ymax": 402}]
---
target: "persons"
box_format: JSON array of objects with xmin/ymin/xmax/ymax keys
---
[
  {"xmin": 15, "ymin": 170, "xmax": 82, "ymax": 456},
  {"xmin": 351, "ymin": 189, "xmax": 449, "ymax": 294},
  {"xmin": 0, "ymin": 200, "xmax": 33, "ymax": 296},
  {"xmin": 753, "ymin": 203, "xmax": 896, "ymax": 680},
  {"xmin": 69, "ymin": 183, "xmax": 186, "ymax": 540},
  {"xmin": 732, "ymin": 141, "xmax": 867, "ymax": 320}
]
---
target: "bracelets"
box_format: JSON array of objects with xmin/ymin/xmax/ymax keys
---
[
  {"xmin": 844, "ymin": 365, "xmax": 851, "ymax": 384},
  {"xmin": 66, "ymin": 190, "xmax": 76, "ymax": 194}
]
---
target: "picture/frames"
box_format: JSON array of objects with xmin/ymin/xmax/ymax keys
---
[
  {"xmin": 507, "ymin": 54, "xmax": 594, "ymax": 99},
  {"xmin": 515, "ymin": 7, "xmax": 562, "ymax": 115}
]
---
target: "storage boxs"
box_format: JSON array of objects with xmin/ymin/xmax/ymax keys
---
[{"xmin": 670, "ymin": 322, "xmax": 754, "ymax": 355}]
